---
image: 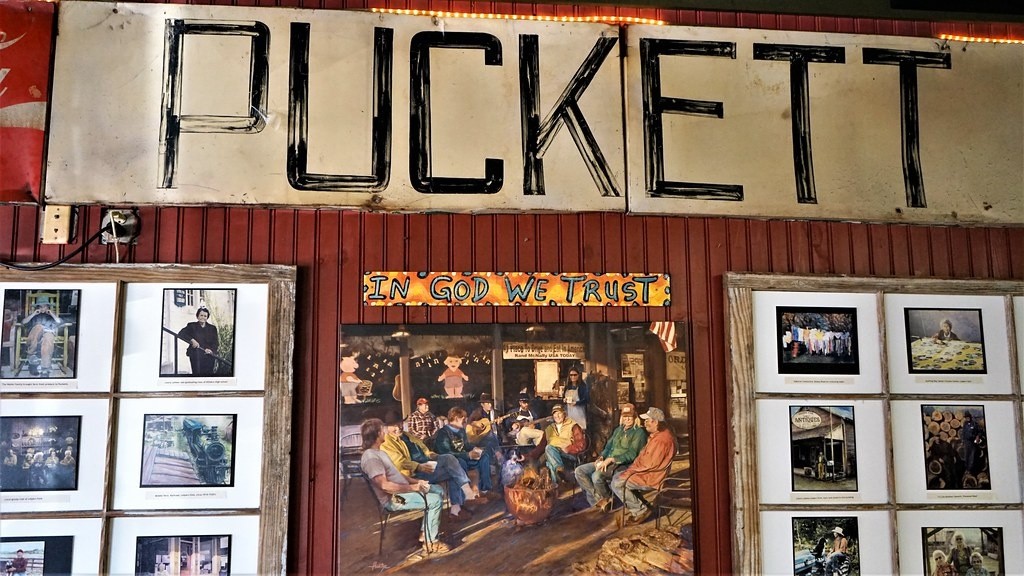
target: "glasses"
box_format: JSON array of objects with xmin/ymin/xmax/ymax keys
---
[
  {"xmin": 935, "ymin": 556, "xmax": 942, "ymax": 560},
  {"xmin": 570, "ymin": 374, "xmax": 579, "ymax": 376},
  {"xmin": 956, "ymin": 538, "xmax": 962, "ymax": 540},
  {"xmin": 972, "ymin": 561, "xmax": 980, "ymax": 563}
]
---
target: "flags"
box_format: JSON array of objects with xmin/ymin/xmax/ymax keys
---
[{"xmin": 648, "ymin": 321, "xmax": 677, "ymax": 353}]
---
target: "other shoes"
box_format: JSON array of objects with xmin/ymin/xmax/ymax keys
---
[
  {"xmin": 627, "ymin": 508, "xmax": 653, "ymax": 526},
  {"xmin": 464, "ymin": 495, "xmax": 488, "ymax": 506},
  {"xmin": 600, "ymin": 497, "xmax": 612, "ymax": 514},
  {"xmin": 38, "ymin": 366, "xmax": 51, "ymax": 378},
  {"xmin": 27, "ymin": 354, "xmax": 41, "ymax": 365},
  {"xmin": 422, "ymin": 540, "xmax": 450, "ymax": 553},
  {"xmin": 418, "ymin": 529, "xmax": 447, "ymax": 542},
  {"xmin": 449, "ymin": 508, "xmax": 472, "ymax": 522}
]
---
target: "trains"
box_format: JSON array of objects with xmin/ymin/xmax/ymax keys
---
[{"xmin": 183, "ymin": 418, "xmax": 231, "ymax": 485}]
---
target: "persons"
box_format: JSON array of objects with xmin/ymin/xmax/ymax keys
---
[
  {"xmin": 933, "ymin": 533, "xmax": 991, "ymax": 576},
  {"xmin": 825, "ymin": 527, "xmax": 847, "ymax": 576},
  {"xmin": 575, "ymin": 402, "xmax": 674, "ymax": 525},
  {"xmin": 933, "ymin": 320, "xmax": 959, "ymax": 340},
  {"xmin": 562, "ymin": 367, "xmax": 588, "ymax": 429},
  {"xmin": 178, "ymin": 308, "xmax": 219, "ymax": 374},
  {"xmin": 408, "ymin": 394, "xmax": 542, "ymax": 513},
  {"xmin": 517, "ymin": 404, "xmax": 587, "ymax": 500},
  {"xmin": 379, "ymin": 410, "xmax": 489, "ymax": 522},
  {"xmin": 818, "ymin": 452, "xmax": 827, "ymax": 480},
  {"xmin": 21, "ymin": 297, "xmax": 60, "ymax": 377},
  {"xmin": 360, "ymin": 419, "xmax": 451, "ymax": 554},
  {"xmin": 6, "ymin": 549, "xmax": 27, "ymax": 576}
]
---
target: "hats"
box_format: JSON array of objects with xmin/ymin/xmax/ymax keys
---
[
  {"xmin": 831, "ymin": 526, "xmax": 845, "ymax": 537},
  {"xmin": 416, "ymin": 397, "xmax": 430, "ymax": 405},
  {"xmin": 551, "ymin": 403, "xmax": 566, "ymax": 415},
  {"xmin": 621, "ymin": 403, "xmax": 635, "ymax": 417},
  {"xmin": 516, "ymin": 393, "xmax": 531, "ymax": 401},
  {"xmin": 639, "ymin": 406, "xmax": 666, "ymax": 422},
  {"xmin": 477, "ymin": 393, "xmax": 494, "ymax": 403},
  {"xmin": 30, "ymin": 295, "xmax": 52, "ymax": 307}
]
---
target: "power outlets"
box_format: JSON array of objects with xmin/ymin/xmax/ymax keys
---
[{"xmin": 42, "ymin": 204, "xmax": 73, "ymax": 244}]
